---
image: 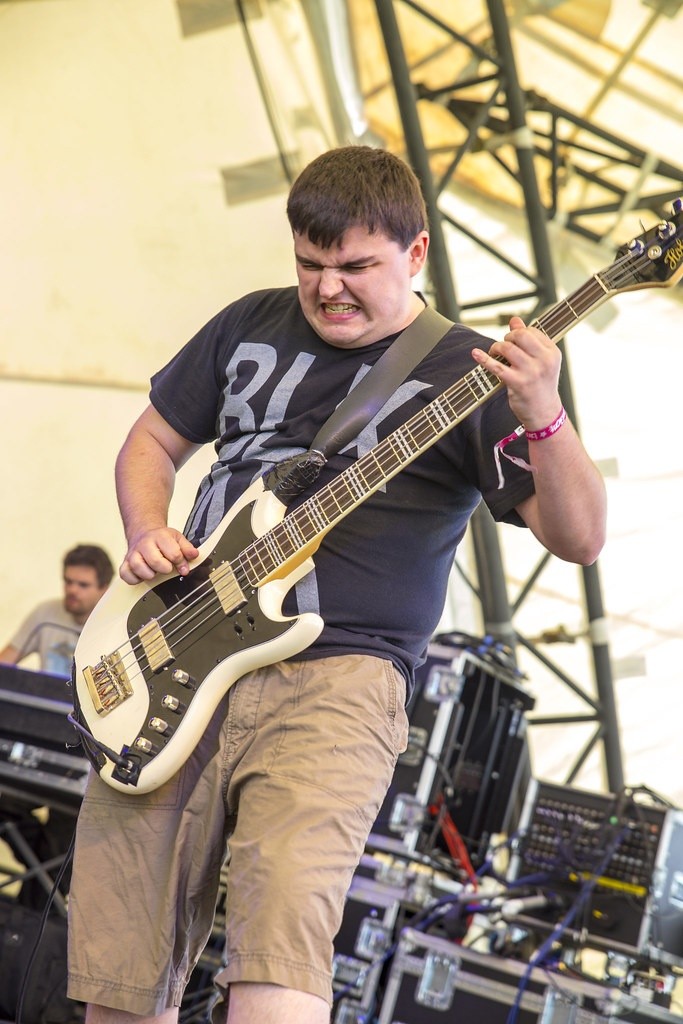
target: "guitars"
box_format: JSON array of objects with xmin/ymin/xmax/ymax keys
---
[{"xmin": 71, "ymin": 198, "xmax": 683, "ymax": 795}]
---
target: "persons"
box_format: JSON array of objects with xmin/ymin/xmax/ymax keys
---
[
  {"xmin": 0, "ymin": 545, "xmax": 115, "ymax": 918},
  {"xmin": 69, "ymin": 145, "xmax": 608, "ymax": 1024}
]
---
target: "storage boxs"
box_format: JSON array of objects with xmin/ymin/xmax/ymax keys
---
[
  {"xmin": 350, "ymin": 854, "xmax": 464, "ymax": 913},
  {"xmin": 377, "ymin": 926, "xmax": 683, "ymax": 1024},
  {"xmin": 0, "ymin": 737, "xmax": 92, "ymax": 798},
  {"xmin": 332, "ymin": 885, "xmax": 425, "ymax": 1012}
]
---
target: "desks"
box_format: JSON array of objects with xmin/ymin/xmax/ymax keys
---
[{"xmin": 0, "ymin": 784, "xmax": 81, "ymax": 921}]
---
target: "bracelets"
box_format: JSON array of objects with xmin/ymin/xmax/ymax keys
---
[{"xmin": 495, "ymin": 407, "xmax": 566, "ymax": 489}]
